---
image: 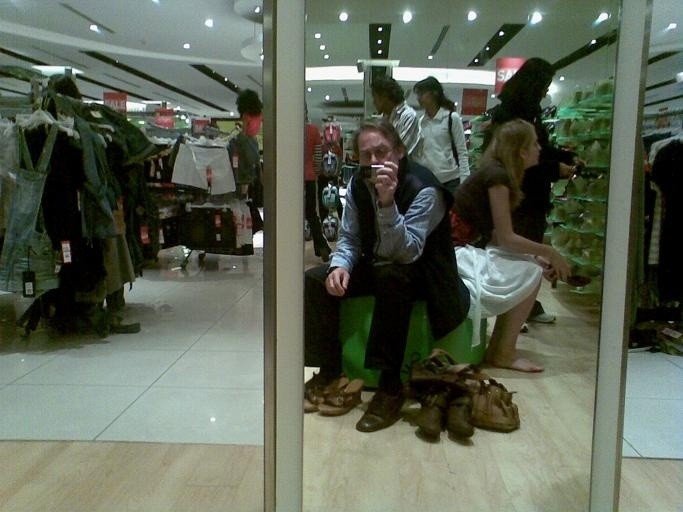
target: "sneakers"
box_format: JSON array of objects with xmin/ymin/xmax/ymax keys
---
[
  {"xmin": 520, "ymin": 322, "xmax": 528, "ymax": 332},
  {"xmin": 529, "ymin": 314, "xmax": 555, "ymax": 323},
  {"xmin": 628, "ymin": 339, "xmax": 653, "ymax": 352}
]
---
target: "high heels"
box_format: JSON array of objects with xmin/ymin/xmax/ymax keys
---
[
  {"xmin": 318, "ymin": 379, "xmax": 363, "ymax": 415},
  {"xmin": 304, "ymin": 376, "xmax": 349, "ymax": 413},
  {"xmin": 549, "ymin": 264, "xmax": 592, "ymax": 288}
]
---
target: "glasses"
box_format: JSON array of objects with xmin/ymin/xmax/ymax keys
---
[{"xmin": 360, "ymin": 148, "xmax": 394, "ymax": 158}]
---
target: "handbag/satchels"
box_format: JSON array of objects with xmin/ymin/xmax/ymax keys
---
[{"xmin": 427, "ymin": 349, "xmax": 520, "ymax": 433}]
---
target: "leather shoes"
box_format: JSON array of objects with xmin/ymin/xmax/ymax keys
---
[
  {"xmin": 357, "ymin": 392, "xmax": 402, "ymax": 432},
  {"xmin": 306, "ymin": 371, "xmax": 344, "ymax": 388}
]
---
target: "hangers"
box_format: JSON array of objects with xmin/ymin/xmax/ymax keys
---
[
  {"xmin": 2, "ymin": 94, "xmax": 115, "ymax": 151},
  {"xmin": 638, "ymin": 108, "xmax": 683, "ymax": 157}
]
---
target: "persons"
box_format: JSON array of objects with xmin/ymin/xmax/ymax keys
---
[
  {"xmin": 484, "ymin": 56, "xmax": 588, "ymax": 333},
  {"xmin": 304, "ymin": 116, "xmax": 472, "ymax": 433},
  {"xmin": 449, "ymin": 118, "xmax": 574, "ymax": 374},
  {"xmin": 370, "ymin": 72, "xmax": 425, "ymax": 165},
  {"xmin": 303, "ymin": 100, "xmax": 332, "ymax": 262},
  {"xmin": 413, "ymin": 75, "xmax": 471, "ymax": 196}
]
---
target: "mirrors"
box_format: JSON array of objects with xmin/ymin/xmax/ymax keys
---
[{"xmin": 261, "ymin": 0, "xmax": 650, "ymax": 512}]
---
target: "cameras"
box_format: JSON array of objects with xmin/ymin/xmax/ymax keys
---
[{"xmin": 358, "ymin": 163, "xmax": 384, "ymax": 183}]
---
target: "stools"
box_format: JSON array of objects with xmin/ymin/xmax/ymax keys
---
[{"xmin": 338, "ymin": 294, "xmax": 489, "ymax": 391}]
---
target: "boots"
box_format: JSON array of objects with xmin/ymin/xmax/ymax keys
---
[
  {"xmin": 411, "ymin": 368, "xmax": 475, "ymax": 437},
  {"xmin": 414, "ymin": 385, "xmax": 449, "ymax": 438}
]
